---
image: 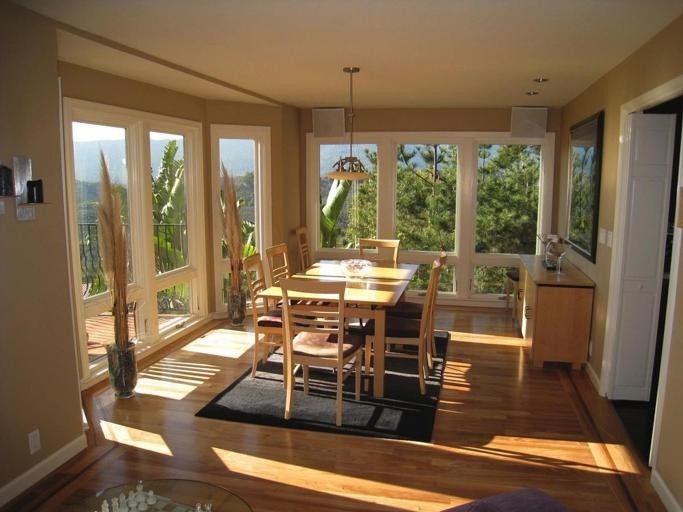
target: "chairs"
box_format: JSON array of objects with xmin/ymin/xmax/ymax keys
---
[
  {"xmin": 241, "ymin": 253, "xmax": 305, "ymax": 381},
  {"xmin": 273, "ymin": 279, "xmax": 362, "ymax": 429},
  {"xmin": 228, "ymin": 235, "xmax": 448, "ymax": 401}
]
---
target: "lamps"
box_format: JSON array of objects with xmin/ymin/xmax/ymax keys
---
[{"xmin": 327, "ymin": 67, "xmax": 371, "ymax": 181}]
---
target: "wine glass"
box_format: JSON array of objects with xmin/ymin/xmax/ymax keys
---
[
  {"xmin": 548, "ymin": 244, "xmax": 572, "ymax": 277},
  {"xmin": 537, "ymin": 232, "xmax": 560, "ymax": 262}
]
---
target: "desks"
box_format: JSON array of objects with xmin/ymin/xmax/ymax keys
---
[{"xmin": 514, "ymin": 252, "xmax": 597, "ymax": 371}]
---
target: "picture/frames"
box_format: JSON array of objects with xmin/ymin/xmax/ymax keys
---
[{"xmin": 565, "ymin": 109, "xmax": 604, "ymax": 265}]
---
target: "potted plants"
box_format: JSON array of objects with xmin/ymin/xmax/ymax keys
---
[
  {"xmin": 215, "ymin": 157, "xmax": 245, "ymax": 324},
  {"xmin": 92, "ymin": 144, "xmax": 138, "ymax": 398}
]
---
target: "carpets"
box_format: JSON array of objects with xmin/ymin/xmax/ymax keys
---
[{"xmin": 195, "ymin": 326, "xmax": 449, "ymax": 443}]
